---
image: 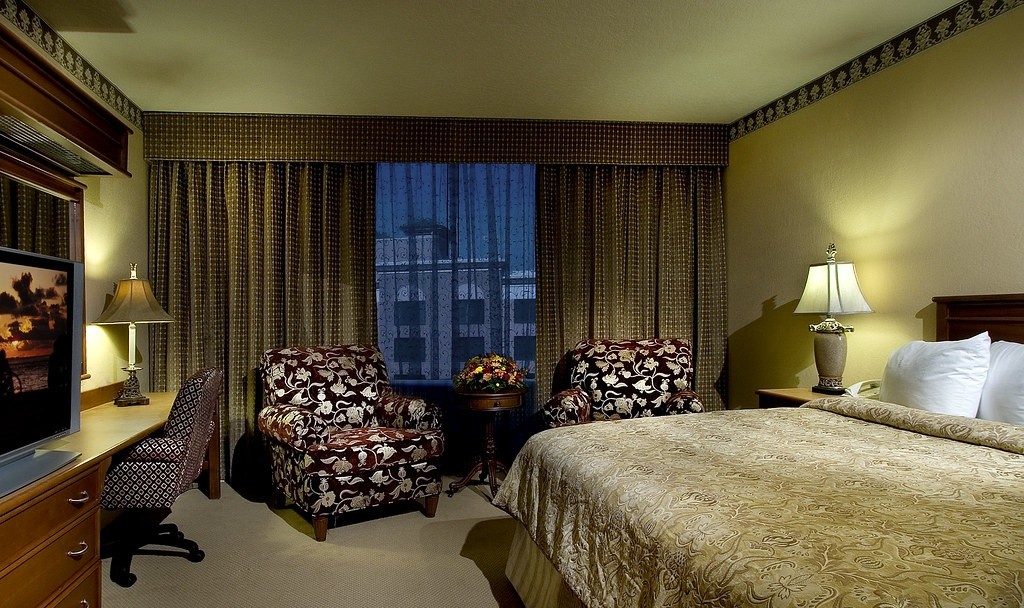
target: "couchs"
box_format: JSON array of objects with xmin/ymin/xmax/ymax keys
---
[
  {"xmin": 258, "ymin": 344, "xmax": 445, "ymax": 542},
  {"xmin": 542, "ymin": 338, "xmax": 706, "ymax": 428}
]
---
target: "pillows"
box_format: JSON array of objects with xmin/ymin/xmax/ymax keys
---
[
  {"xmin": 976, "ymin": 340, "xmax": 1024, "ymax": 426},
  {"xmin": 879, "ymin": 332, "xmax": 992, "ymax": 418}
]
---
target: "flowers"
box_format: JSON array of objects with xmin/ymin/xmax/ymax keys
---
[{"xmin": 453, "ymin": 352, "xmax": 530, "ymax": 393}]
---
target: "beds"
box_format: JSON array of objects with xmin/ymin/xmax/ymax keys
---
[{"xmin": 493, "ymin": 293, "xmax": 1024, "ymax": 608}]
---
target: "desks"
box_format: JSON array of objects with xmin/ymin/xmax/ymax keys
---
[{"xmin": 0, "ymin": 381, "xmax": 220, "ymax": 608}]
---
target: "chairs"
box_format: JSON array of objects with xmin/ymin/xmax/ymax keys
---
[
  {"xmin": 0, "ymin": 349, "xmax": 22, "ymax": 394},
  {"xmin": 99, "ymin": 366, "xmax": 223, "ymax": 588}
]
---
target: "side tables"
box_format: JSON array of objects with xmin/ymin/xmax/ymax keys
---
[{"xmin": 448, "ymin": 390, "xmax": 527, "ymax": 498}]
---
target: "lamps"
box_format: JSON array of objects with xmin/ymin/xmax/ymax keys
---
[
  {"xmin": 91, "ymin": 263, "xmax": 176, "ymax": 407},
  {"xmin": 792, "ymin": 242, "xmax": 876, "ymax": 395}
]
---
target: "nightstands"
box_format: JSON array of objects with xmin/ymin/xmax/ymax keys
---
[{"xmin": 756, "ymin": 387, "xmax": 842, "ymax": 409}]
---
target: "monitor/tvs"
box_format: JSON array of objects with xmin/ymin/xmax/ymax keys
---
[{"xmin": 0, "ymin": 245, "xmax": 84, "ymax": 497}]
